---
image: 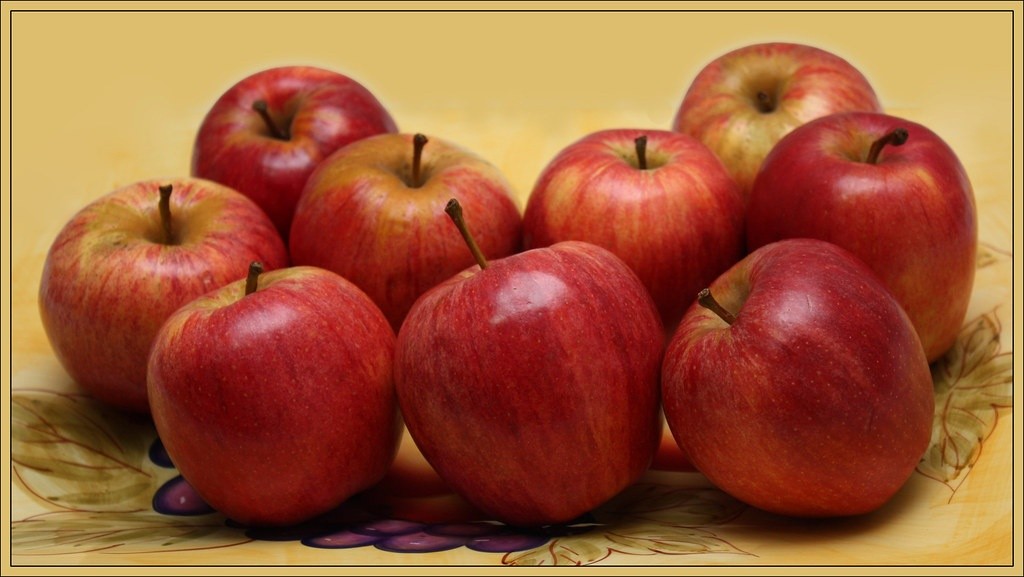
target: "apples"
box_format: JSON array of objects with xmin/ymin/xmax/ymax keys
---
[
  {"xmin": 37, "ymin": 177, "xmax": 289, "ymax": 416},
  {"xmin": 392, "ymin": 198, "xmax": 669, "ymax": 527},
  {"xmin": 519, "ymin": 128, "xmax": 743, "ymax": 339},
  {"xmin": 672, "ymin": 42, "xmax": 880, "ymax": 198},
  {"xmin": 288, "ymin": 131, "xmax": 521, "ymax": 336},
  {"xmin": 660, "ymin": 238, "xmax": 935, "ymax": 518},
  {"xmin": 147, "ymin": 262, "xmax": 407, "ymax": 526},
  {"xmin": 743, "ymin": 112, "xmax": 979, "ymax": 363},
  {"xmin": 191, "ymin": 65, "xmax": 398, "ymax": 246}
]
